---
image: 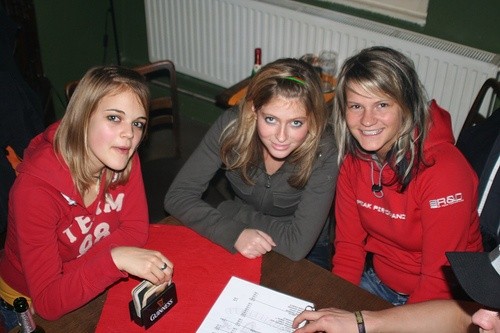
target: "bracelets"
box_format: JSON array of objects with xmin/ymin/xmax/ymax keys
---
[{"xmin": 354, "ymin": 310, "xmax": 365, "ymax": 333}]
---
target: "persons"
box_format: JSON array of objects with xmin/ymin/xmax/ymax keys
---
[
  {"xmin": 0, "ymin": 65, "xmax": 173, "ymax": 328},
  {"xmin": 327, "ymin": 46, "xmax": 484, "ymax": 307},
  {"xmin": 290, "ymin": 245, "xmax": 500, "ymax": 333},
  {"xmin": 163, "ymin": 57, "xmax": 337, "ymax": 268}
]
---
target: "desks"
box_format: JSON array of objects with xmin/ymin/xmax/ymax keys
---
[
  {"xmin": 9, "ymin": 216, "xmax": 395, "ymax": 333},
  {"xmin": 215, "ymin": 73, "xmax": 337, "ymax": 107}
]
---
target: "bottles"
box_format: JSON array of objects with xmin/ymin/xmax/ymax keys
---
[
  {"xmin": 251, "ymin": 48, "xmax": 262, "ymax": 75},
  {"xmin": 13, "ymin": 297, "xmax": 46, "ymax": 333}
]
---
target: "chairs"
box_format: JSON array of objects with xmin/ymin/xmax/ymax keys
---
[{"xmin": 65, "ymin": 60, "xmax": 180, "ymax": 222}]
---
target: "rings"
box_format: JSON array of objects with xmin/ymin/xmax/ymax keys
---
[{"xmin": 161, "ymin": 264, "xmax": 167, "ymax": 271}]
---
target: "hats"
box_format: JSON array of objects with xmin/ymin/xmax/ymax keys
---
[{"xmin": 446, "ymin": 245, "xmax": 500, "ymax": 309}]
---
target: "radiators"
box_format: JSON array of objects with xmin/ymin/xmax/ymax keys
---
[{"xmin": 144, "ymin": 0, "xmax": 500, "ymax": 141}]
